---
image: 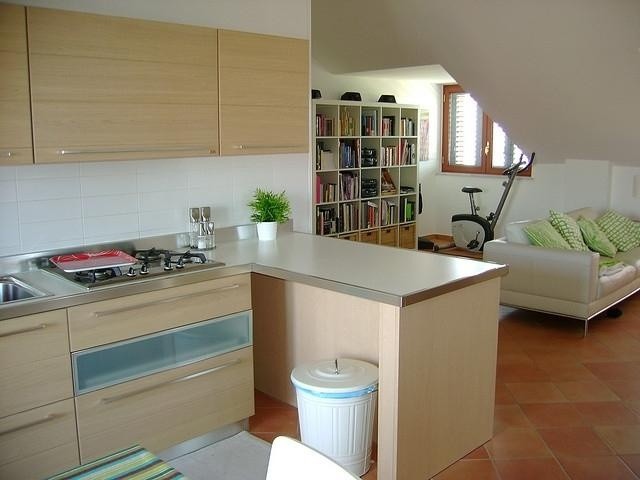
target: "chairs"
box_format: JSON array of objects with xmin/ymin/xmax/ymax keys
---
[{"xmin": 266, "ymin": 436, "xmax": 362, "ymax": 480}]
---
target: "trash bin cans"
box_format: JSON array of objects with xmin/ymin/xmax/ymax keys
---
[{"xmin": 290, "ymin": 356, "xmax": 378, "ymax": 477}]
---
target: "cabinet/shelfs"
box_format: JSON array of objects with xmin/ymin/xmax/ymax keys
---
[
  {"xmin": 67, "ymin": 269, "xmax": 255, "ymax": 467},
  {"xmin": 26, "ymin": 4, "xmax": 218, "ymax": 165},
  {"xmin": 0, "ymin": 309, "xmax": 80, "ymax": 480},
  {"xmin": 312, "ymin": 98, "xmax": 420, "ymax": 251},
  {"xmin": 0, "ymin": 2, "xmax": 35, "ymax": 166},
  {"xmin": 217, "ymin": 27, "xmax": 309, "ymax": 156}
]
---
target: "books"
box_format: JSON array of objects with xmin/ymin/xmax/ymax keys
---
[
  {"xmin": 317, "ymin": 143, "xmax": 336, "ymax": 170},
  {"xmin": 315, "ymin": 115, "xmax": 336, "ymax": 136},
  {"xmin": 381, "ymin": 170, "xmax": 396, "ymax": 193},
  {"xmin": 340, "ymin": 205, "xmax": 359, "ymax": 232},
  {"xmin": 401, "ymin": 198, "xmax": 416, "ymax": 222},
  {"xmin": 363, "ymin": 110, "xmax": 377, "ymax": 136},
  {"xmin": 339, "ymin": 109, "xmax": 357, "ymax": 136},
  {"xmin": 382, "ymin": 116, "xmax": 396, "ymax": 136},
  {"xmin": 363, "ymin": 202, "xmax": 380, "ymax": 227},
  {"xmin": 402, "ymin": 118, "xmax": 415, "ymax": 135},
  {"xmin": 317, "ymin": 207, "xmax": 337, "ymax": 234},
  {"xmin": 382, "ymin": 201, "xmax": 398, "ymax": 226},
  {"xmin": 317, "ymin": 177, "xmax": 338, "ymax": 203},
  {"xmin": 339, "ymin": 173, "xmax": 358, "ymax": 200},
  {"xmin": 340, "ymin": 142, "xmax": 358, "ymax": 168},
  {"xmin": 401, "ymin": 139, "xmax": 416, "ymax": 165},
  {"xmin": 401, "ymin": 187, "xmax": 416, "ymax": 192},
  {"xmin": 382, "ymin": 146, "xmax": 398, "ymax": 166}
]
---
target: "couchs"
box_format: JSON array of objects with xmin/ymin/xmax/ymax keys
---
[{"xmin": 483, "ymin": 206, "xmax": 640, "ymax": 337}]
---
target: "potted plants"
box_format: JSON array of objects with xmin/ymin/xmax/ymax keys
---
[{"xmin": 247, "ymin": 186, "xmax": 291, "ymax": 241}]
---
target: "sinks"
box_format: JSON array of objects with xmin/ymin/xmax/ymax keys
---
[{"xmin": 0, "ymin": 275, "xmax": 54, "ymax": 305}]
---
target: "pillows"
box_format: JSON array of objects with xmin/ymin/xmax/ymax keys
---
[
  {"xmin": 595, "ymin": 209, "xmax": 640, "ymax": 253},
  {"xmin": 522, "ymin": 219, "xmax": 569, "ymax": 249},
  {"xmin": 576, "ymin": 214, "xmax": 617, "ymax": 258},
  {"xmin": 549, "ymin": 207, "xmax": 591, "ymax": 252}
]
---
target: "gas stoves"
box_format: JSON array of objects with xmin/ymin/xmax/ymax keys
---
[{"xmin": 40, "ymin": 247, "xmax": 225, "ymax": 291}]
